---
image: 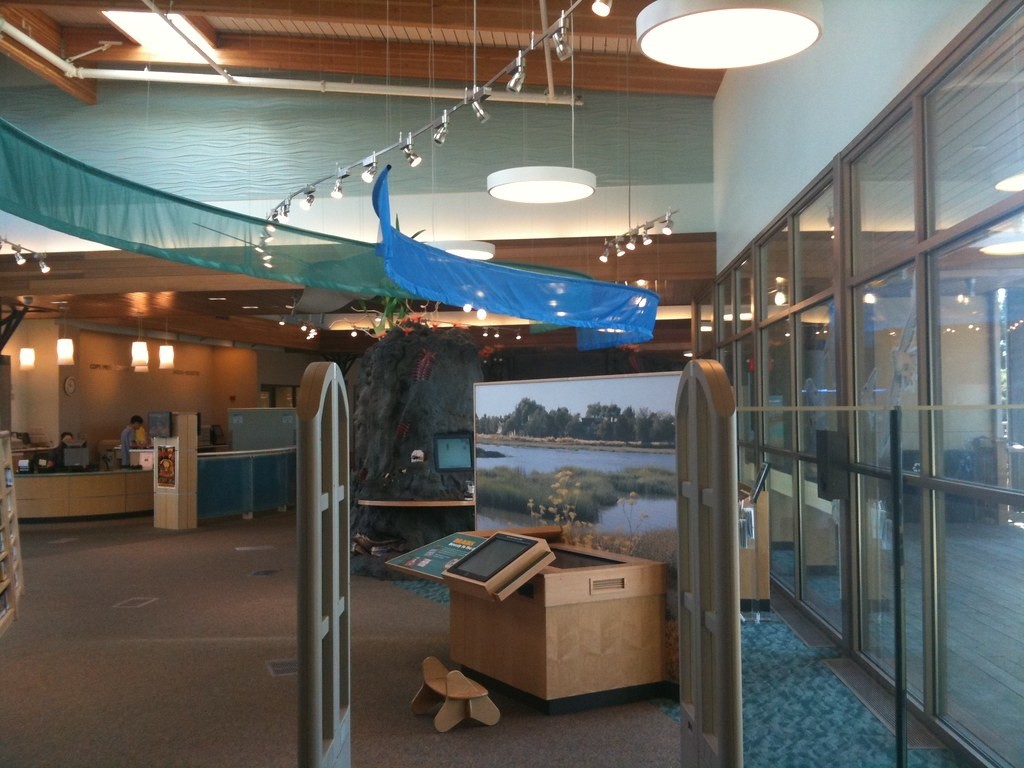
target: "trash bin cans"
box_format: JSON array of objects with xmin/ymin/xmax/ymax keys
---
[
  {"xmin": 944, "ymin": 450, "xmax": 979, "ymax": 522},
  {"xmin": 901, "ymin": 450, "xmax": 923, "ymax": 521}
]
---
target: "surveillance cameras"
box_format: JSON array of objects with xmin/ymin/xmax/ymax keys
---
[{"xmin": 24, "ymin": 296, "xmax": 33, "ymax": 305}]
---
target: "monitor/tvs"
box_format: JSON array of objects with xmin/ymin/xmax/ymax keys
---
[
  {"xmin": 433, "ymin": 434, "xmax": 474, "ymax": 473},
  {"xmin": 61, "ymin": 440, "xmax": 87, "ymax": 471},
  {"xmin": 447, "ymin": 533, "xmax": 538, "ymax": 582},
  {"xmin": 147, "ymin": 411, "xmax": 172, "ymax": 438},
  {"xmin": 212, "ymin": 425, "xmax": 224, "ymax": 445}
]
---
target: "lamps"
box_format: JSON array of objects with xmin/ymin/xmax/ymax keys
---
[
  {"xmin": 361, "ymin": 157, "xmax": 380, "ymax": 183},
  {"xmin": 19, "ymin": 316, "xmax": 36, "ymax": 372},
  {"xmin": 635, "ymin": 0, "xmax": 824, "ymax": 71},
  {"xmin": 422, "ymin": 240, "xmax": 496, "ymax": 261},
  {"xmin": 255, "ymin": 184, "xmax": 317, "ymax": 268},
  {"xmin": 98, "ymin": 9, "xmax": 219, "ymax": 50},
  {"xmin": 279, "ymin": 312, "xmax": 521, "ymax": 341},
  {"xmin": 159, "ymin": 318, "xmax": 175, "ymax": 370},
  {"xmin": 471, "ymin": 87, "xmax": 493, "ymax": 123},
  {"xmin": 827, "ymin": 206, "xmax": 836, "ymax": 239},
  {"xmin": 12, "ymin": 246, "xmax": 26, "ymax": 265},
  {"xmin": 398, "ymin": 138, "xmax": 422, "ymax": 167},
  {"xmin": 701, "ymin": 322, "xmax": 712, "ymax": 331},
  {"xmin": 432, "ymin": 116, "xmax": 450, "ymax": 145},
  {"xmin": 330, "ymin": 168, "xmax": 351, "ymax": 199},
  {"xmin": 34, "ymin": 253, "xmax": 51, "ymax": 273},
  {"xmin": 134, "ymin": 313, "xmax": 149, "ymax": 373},
  {"xmin": 591, "ymin": 0, "xmax": 613, "ymax": 17},
  {"xmin": 599, "ymin": 210, "xmax": 678, "ymax": 263},
  {"xmin": 55, "ymin": 305, "xmax": 75, "ymax": 366},
  {"xmin": 683, "ymin": 350, "xmax": 692, "ymax": 357},
  {"xmin": 487, "ymin": 0, "xmax": 598, "ymax": 204},
  {"xmin": 505, "ymin": 57, "xmax": 528, "ymax": 94},
  {"xmin": 547, "ymin": 17, "xmax": 573, "ymax": 62},
  {"xmin": 131, "ymin": 312, "xmax": 148, "ymax": 367}
]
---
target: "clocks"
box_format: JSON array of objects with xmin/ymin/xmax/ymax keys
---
[{"xmin": 64, "ymin": 376, "xmax": 78, "ymax": 397}]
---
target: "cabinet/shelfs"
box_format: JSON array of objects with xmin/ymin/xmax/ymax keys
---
[
  {"xmin": 737, "ymin": 483, "xmax": 772, "ymax": 621},
  {"xmin": 0, "ymin": 430, "xmax": 26, "ymax": 638}
]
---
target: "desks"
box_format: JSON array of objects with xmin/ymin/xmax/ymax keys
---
[
  {"xmin": 12, "ymin": 447, "xmax": 58, "ymax": 470},
  {"xmin": 981, "ymin": 438, "xmax": 1024, "ymax": 513},
  {"xmin": 359, "ymin": 499, "xmax": 476, "ymax": 508},
  {"xmin": 902, "ymin": 448, "xmax": 975, "ymax": 523},
  {"xmin": 386, "ymin": 525, "xmax": 670, "ymax": 715}
]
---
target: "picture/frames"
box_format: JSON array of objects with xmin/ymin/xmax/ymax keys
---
[{"xmin": 473, "ymin": 371, "xmax": 683, "ymax": 621}]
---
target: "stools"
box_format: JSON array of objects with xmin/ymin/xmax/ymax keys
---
[{"xmin": 410, "ymin": 656, "xmax": 501, "ymax": 732}]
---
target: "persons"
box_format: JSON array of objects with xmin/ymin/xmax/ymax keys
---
[
  {"xmin": 121, "ymin": 414, "xmax": 143, "ymax": 466},
  {"xmin": 45, "ymin": 432, "xmax": 74, "ymax": 468}
]
